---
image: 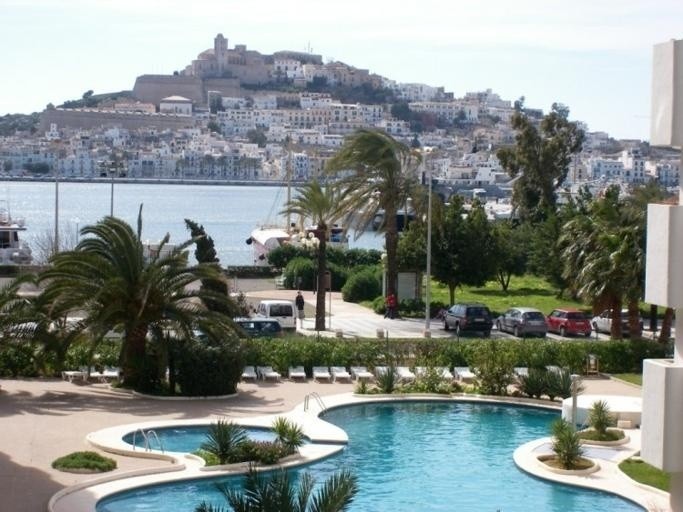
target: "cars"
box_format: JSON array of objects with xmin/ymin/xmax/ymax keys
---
[
  {"xmin": 194, "ymin": 300, "xmax": 297, "ymax": 338},
  {"xmin": 442, "ymin": 301, "xmax": 644, "ymax": 337}
]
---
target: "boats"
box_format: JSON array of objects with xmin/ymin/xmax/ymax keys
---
[
  {"xmin": 251, "ymin": 128, "xmax": 349, "ymax": 252},
  {"xmin": 0, "ymin": 198, "xmax": 34, "ymax": 274},
  {"xmin": 341, "ymin": 206, "xmax": 416, "ymax": 232}
]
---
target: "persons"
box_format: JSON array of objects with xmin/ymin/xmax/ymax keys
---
[
  {"xmin": 384, "ymin": 294, "xmax": 395, "ymax": 319},
  {"xmin": 296, "ymin": 292, "xmax": 304, "ymax": 310}
]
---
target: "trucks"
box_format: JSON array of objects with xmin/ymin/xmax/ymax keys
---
[{"xmin": 458, "ymin": 189, "xmax": 487, "ymax": 204}]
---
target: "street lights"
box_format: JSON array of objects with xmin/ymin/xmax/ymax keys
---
[
  {"xmin": 422, "ymin": 145, "xmax": 439, "ymax": 339},
  {"xmin": 50, "ymin": 137, "xmax": 62, "ymax": 256},
  {"xmin": 96, "ymin": 160, "xmax": 127, "ymax": 215}
]
